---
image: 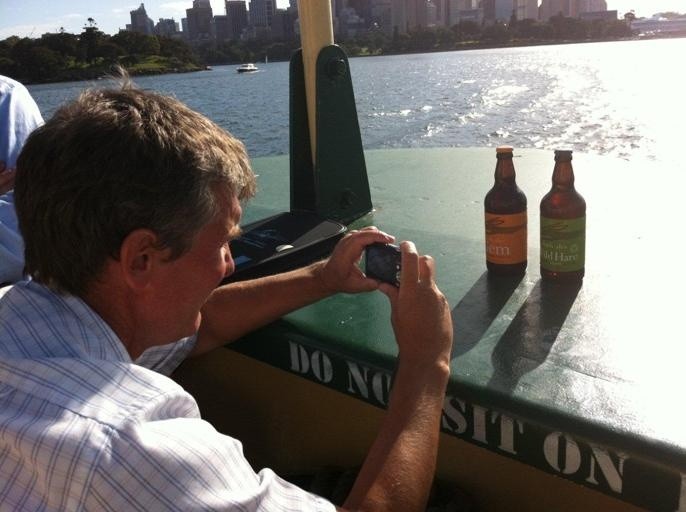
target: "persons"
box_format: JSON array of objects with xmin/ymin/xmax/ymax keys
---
[
  {"xmin": 0, "ymin": 74, "xmax": 46, "ymax": 288},
  {"xmin": 0, "ymin": 89, "xmax": 453, "ymax": 512}
]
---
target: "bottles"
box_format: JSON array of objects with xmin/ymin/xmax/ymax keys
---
[{"xmin": 483, "ymin": 145, "xmax": 587, "ymax": 282}]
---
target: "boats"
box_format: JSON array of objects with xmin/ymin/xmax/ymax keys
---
[{"xmin": 236, "ymin": 63, "xmax": 257, "ymax": 73}]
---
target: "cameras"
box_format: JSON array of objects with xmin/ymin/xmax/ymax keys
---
[{"xmin": 364, "ymin": 242, "xmax": 402, "ymax": 289}]
